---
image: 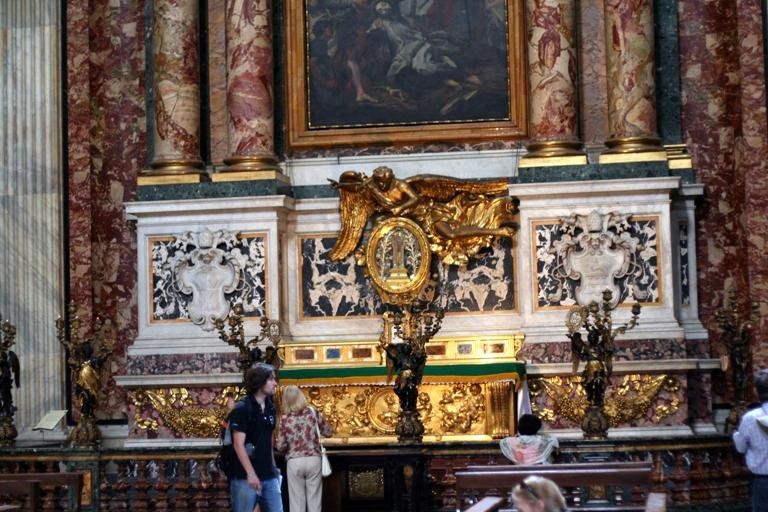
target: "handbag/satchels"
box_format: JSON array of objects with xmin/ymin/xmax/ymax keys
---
[{"xmin": 321, "ymin": 448, "xmax": 331, "ymax": 477}]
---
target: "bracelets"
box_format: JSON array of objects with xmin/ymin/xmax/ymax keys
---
[{"xmin": 246, "ymin": 471, "xmax": 255, "ymax": 477}]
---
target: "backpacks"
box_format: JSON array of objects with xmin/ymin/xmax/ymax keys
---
[{"xmin": 215, "ymin": 397, "xmax": 248, "ymax": 475}]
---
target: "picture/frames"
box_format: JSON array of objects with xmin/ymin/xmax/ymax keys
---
[{"xmin": 269, "ymin": 0, "xmax": 530, "ymax": 150}]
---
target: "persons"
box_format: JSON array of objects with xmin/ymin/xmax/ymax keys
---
[
  {"xmin": 579, "ymin": 330, "xmax": 606, "ymax": 405},
  {"xmin": 498, "ymin": 415, "xmax": 560, "ymax": 465},
  {"xmin": 731, "ymin": 368, "xmax": 768, "ymax": 512},
  {"xmin": 221, "ymin": 361, "xmax": 284, "ymax": 512},
  {"xmin": 382, "ymin": 344, "xmax": 425, "ymax": 410},
  {"xmin": 275, "ymin": 384, "xmax": 334, "ymax": 512},
  {"xmin": 327, "ymin": 166, "xmax": 515, "ymax": 239},
  {"xmin": 510, "ymin": 476, "xmax": 568, "ymax": 512}
]
